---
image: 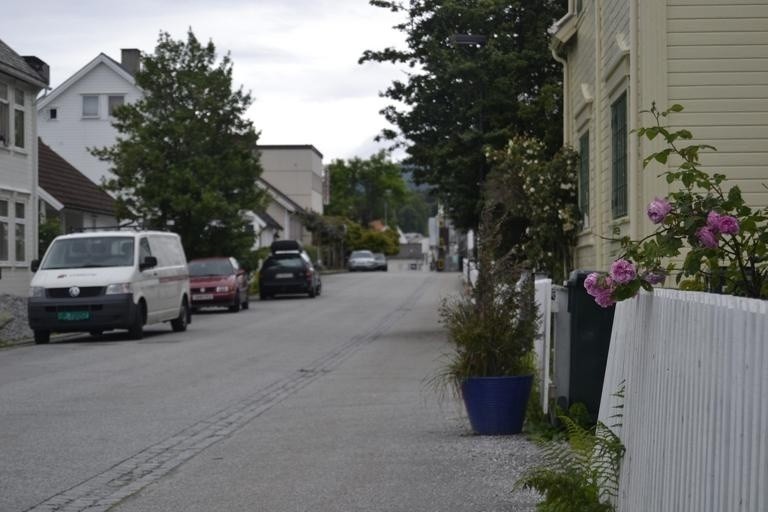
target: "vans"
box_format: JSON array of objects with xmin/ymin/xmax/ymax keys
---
[{"xmin": 27, "ymin": 230, "xmax": 191, "ymax": 344}]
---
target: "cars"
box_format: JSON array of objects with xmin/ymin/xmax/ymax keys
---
[
  {"xmin": 188, "ymin": 253, "xmax": 248, "ymax": 313},
  {"xmin": 259, "ymin": 240, "xmax": 321, "ymax": 298},
  {"xmin": 348, "ymin": 249, "xmax": 387, "ymax": 272}
]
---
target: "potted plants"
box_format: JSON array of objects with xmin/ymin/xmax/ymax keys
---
[{"xmin": 420, "ymin": 179, "xmax": 542, "ymax": 435}]
